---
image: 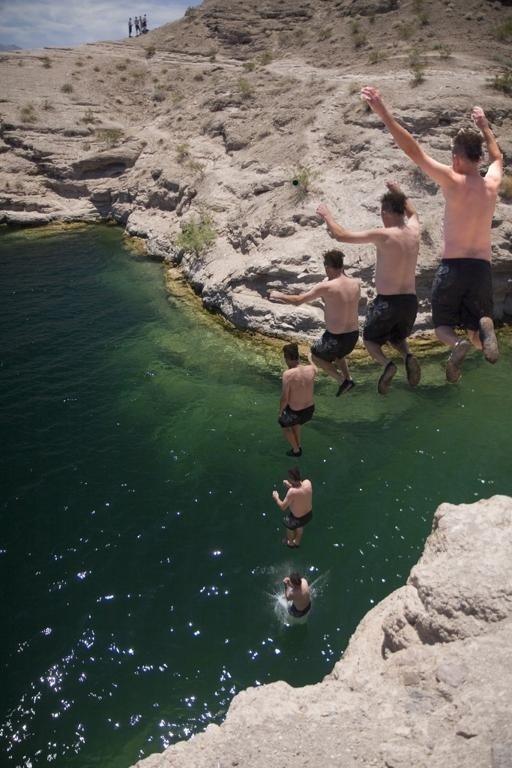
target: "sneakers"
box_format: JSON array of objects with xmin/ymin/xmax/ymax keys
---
[
  {"xmin": 282, "ymin": 538, "xmax": 301, "ymax": 549},
  {"xmin": 406, "ymin": 356, "xmax": 420, "ymax": 385},
  {"xmin": 378, "ymin": 362, "xmax": 396, "ymax": 394},
  {"xmin": 479, "ymin": 317, "xmax": 499, "ymax": 364},
  {"xmin": 446, "ymin": 340, "xmax": 468, "ymax": 383},
  {"xmin": 335, "ymin": 380, "xmax": 355, "ymax": 397},
  {"xmin": 287, "ymin": 447, "xmax": 302, "ymax": 457}
]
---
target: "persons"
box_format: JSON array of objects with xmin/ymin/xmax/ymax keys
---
[
  {"xmin": 361, "ymin": 86, "xmax": 505, "ymax": 384},
  {"xmin": 278, "ymin": 341, "xmax": 318, "ymax": 457},
  {"xmin": 273, "ymin": 467, "xmax": 313, "ymax": 549},
  {"xmin": 317, "ymin": 179, "xmax": 421, "ymax": 393},
  {"xmin": 283, "ymin": 573, "xmax": 312, "ymax": 619},
  {"xmin": 128, "ymin": 14, "xmax": 147, "ymax": 37},
  {"xmin": 269, "ymin": 250, "xmax": 362, "ymax": 396}
]
